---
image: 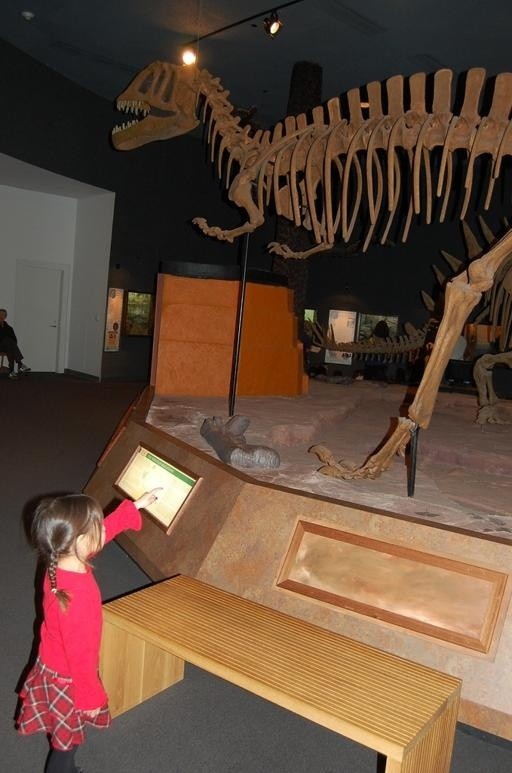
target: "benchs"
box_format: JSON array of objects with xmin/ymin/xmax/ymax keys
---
[{"xmin": 99, "ymin": 571, "xmax": 466, "ymax": 773}]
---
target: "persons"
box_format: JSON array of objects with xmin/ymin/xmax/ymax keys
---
[
  {"xmin": 15, "ymin": 486, "xmax": 166, "ymax": 773},
  {"xmin": 0, "ymin": 309, "xmax": 31, "ymax": 381}
]
